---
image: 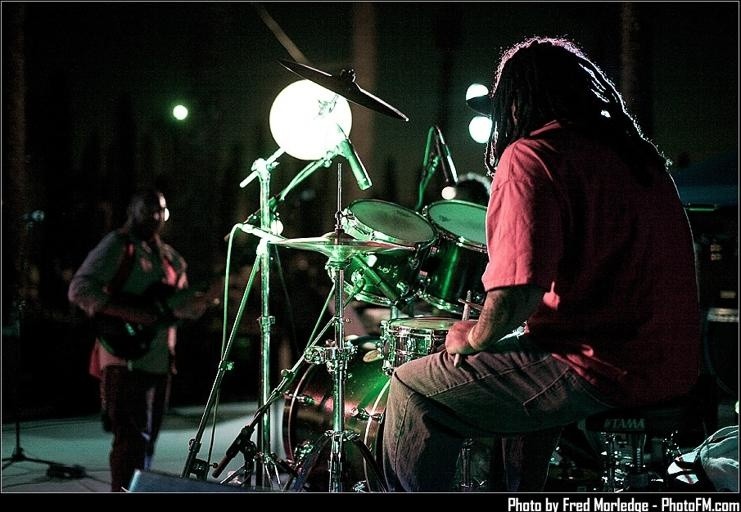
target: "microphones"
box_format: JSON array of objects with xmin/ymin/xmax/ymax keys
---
[
  {"xmin": 435, "ymin": 127, "xmax": 459, "ymax": 185},
  {"xmin": 13, "ymin": 209, "xmax": 45, "ymax": 222},
  {"xmin": 336, "ymin": 125, "xmax": 373, "ymax": 191}
]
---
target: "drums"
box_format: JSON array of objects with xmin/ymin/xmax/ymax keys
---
[
  {"xmin": 382, "ymin": 318, "xmax": 460, "ymax": 374},
  {"xmin": 416, "ymin": 200, "xmax": 487, "ymax": 316},
  {"xmin": 324, "ymin": 199, "xmax": 439, "ymax": 308},
  {"xmin": 281, "ymin": 337, "xmax": 523, "ymax": 494}
]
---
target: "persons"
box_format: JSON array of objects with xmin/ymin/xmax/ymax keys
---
[
  {"xmin": 382, "ymin": 34, "xmax": 701, "ymax": 492},
  {"xmin": 24, "ymin": 252, "xmax": 354, "ymax": 403},
  {"xmin": 66, "ymin": 186, "xmax": 223, "ymax": 493}
]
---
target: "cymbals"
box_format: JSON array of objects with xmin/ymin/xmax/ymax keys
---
[
  {"xmin": 279, "ymin": 59, "xmax": 410, "ymax": 120},
  {"xmin": 469, "ymin": 93, "xmax": 492, "ymax": 119}
]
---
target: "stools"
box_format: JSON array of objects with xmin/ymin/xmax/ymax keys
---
[{"xmin": 588, "ymin": 406, "xmax": 687, "ymax": 494}]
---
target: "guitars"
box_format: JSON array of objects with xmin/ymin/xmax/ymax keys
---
[{"xmin": 96, "ymin": 266, "xmax": 257, "ymax": 360}]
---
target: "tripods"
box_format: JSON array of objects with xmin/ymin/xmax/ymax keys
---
[
  {"xmin": 2, "ymin": 224, "xmax": 87, "ymax": 479},
  {"xmin": 292, "ymin": 269, "xmax": 392, "ymax": 493},
  {"xmin": 220, "ymin": 96, "xmax": 339, "ymax": 492}
]
